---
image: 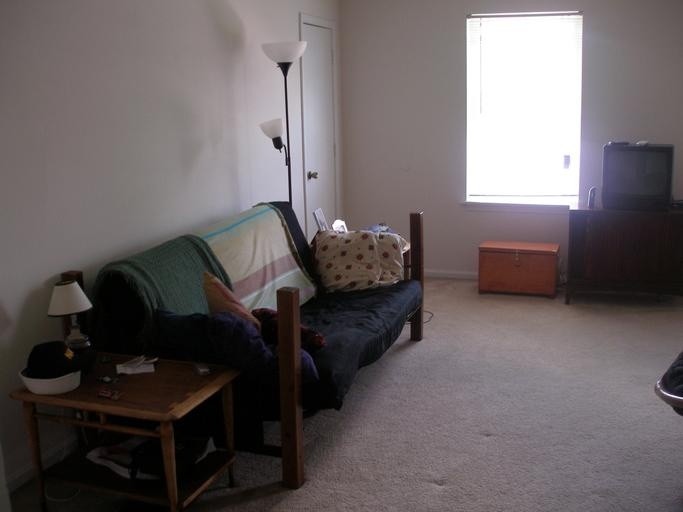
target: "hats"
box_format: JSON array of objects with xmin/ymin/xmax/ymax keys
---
[{"xmin": 27, "ymin": 342, "xmax": 87, "ymax": 378}]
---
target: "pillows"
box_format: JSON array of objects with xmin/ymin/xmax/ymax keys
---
[
  {"xmin": 316, "ymin": 226, "xmax": 410, "ymax": 293},
  {"xmin": 154, "ymin": 270, "xmax": 321, "ymax": 379}
]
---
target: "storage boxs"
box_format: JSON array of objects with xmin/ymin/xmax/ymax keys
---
[{"xmin": 478, "ymin": 240, "xmax": 560, "ymax": 297}]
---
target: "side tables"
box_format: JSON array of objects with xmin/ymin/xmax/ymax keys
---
[{"xmin": 9, "ymin": 353, "xmax": 243, "ymax": 509}]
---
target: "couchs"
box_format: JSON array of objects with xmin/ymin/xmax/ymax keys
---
[{"xmin": 96, "ymin": 202, "xmax": 427, "ymax": 487}]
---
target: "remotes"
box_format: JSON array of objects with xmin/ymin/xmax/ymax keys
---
[{"xmin": 636, "ymin": 140, "xmax": 648, "ymax": 146}]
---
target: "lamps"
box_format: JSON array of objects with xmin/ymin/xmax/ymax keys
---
[
  {"xmin": 49, "ymin": 282, "xmax": 95, "ymax": 349},
  {"xmin": 258, "ymin": 39, "xmax": 308, "ymax": 207}
]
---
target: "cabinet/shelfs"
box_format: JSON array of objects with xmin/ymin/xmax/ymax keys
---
[{"xmin": 566, "ymin": 209, "xmax": 683, "ymax": 304}]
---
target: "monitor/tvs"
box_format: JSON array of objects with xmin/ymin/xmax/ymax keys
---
[{"xmin": 601, "ymin": 143, "xmax": 673, "ymax": 211}]
